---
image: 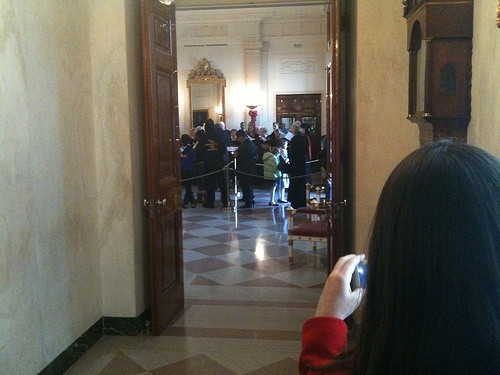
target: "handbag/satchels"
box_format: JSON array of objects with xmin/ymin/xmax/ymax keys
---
[
  {"xmin": 277, "ymin": 156, "xmax": 290, "ymax": 173},
  {"xmin": 282, "ymin": 173, "xmax": 290, "ymax": 189}
]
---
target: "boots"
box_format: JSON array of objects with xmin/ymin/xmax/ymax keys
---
[
  {"xmin": 204, "ymin": 190, "xmax": 208, "ymax": 202},
  {"xmin": 197, "ymin": 188, "xmax": 202, "ymax": 203}
]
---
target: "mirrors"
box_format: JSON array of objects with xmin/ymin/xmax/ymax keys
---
[{"xmin": 187, "ymin": 57, "xmax": 226, "ymax": 130}]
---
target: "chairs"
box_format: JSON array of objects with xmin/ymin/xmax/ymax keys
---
[{"xmin": 286, "ymin": 183, "xmax": 331, "ymax": 270}]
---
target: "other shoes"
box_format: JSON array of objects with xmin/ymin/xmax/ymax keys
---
[
  {"xmin": 183, "ymin": 203, "xmax": 188, "ymax": 208},
  {"xmin": 191, "ymin": 200, "xmax": 196, "ymax": 207},
  {"xmin": 239, "ymin": 198, "xmax": 250, "ymax": 202},
  {"xmin": 269, "ymin": 202, "xmax": 279, "ymax": 206},
  {"xmin": 242, "ymin": 202, "xmax": 255, "ymax": 208},
  {"xmin": 208, "ymin": 201, "xmax": 214, "ymax": 208},
  {"xmin": 278, "ymin": 199, "xmax": 287, "ymax": 203},
  {"xmin": 223, "ymin": 202, "xmax": 227, "ymax": 208}
]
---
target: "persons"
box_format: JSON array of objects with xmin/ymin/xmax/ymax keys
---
[
  {"xmin": 299, "ymin": 139, "xmax": 500, "ymax": 375},
  {"xmin": 179, "ymin": 118, "xmax": 318, "ymax": 211}
]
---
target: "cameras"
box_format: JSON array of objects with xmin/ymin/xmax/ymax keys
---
[{"xmin": 353, "ymin": 263, "xmax": 368, "ymax": 289}]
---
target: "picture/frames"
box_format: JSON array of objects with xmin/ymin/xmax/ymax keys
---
[{"xmin": 193, "ymin": 109, "xmax": 210, "ymax": 128}]
